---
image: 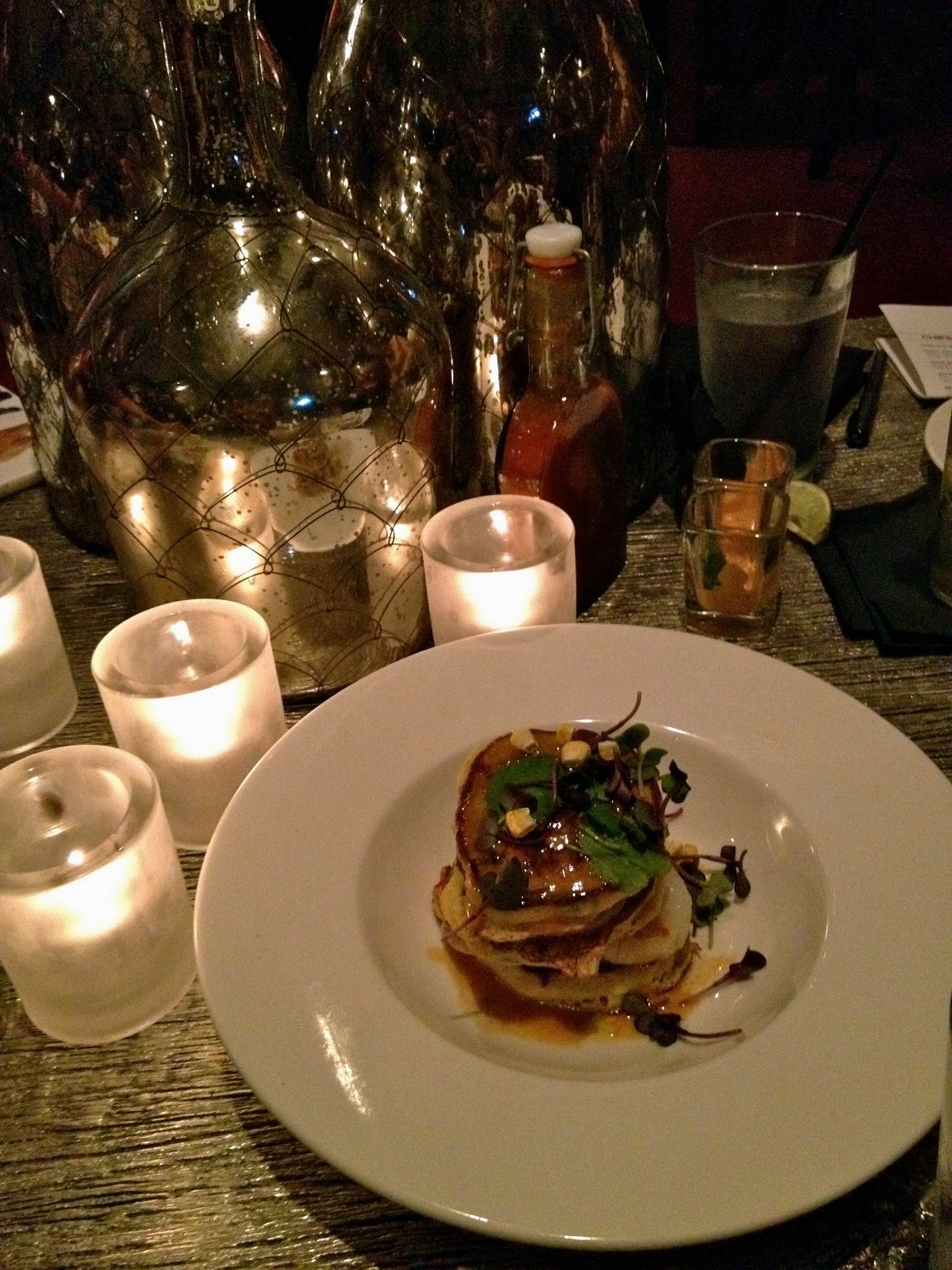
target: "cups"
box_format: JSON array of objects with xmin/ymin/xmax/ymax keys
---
[
  {"xmin": 0, "ymin": 743, "xmax": 194, "ymax": 1047},
  {"xmin": 693, "ymin": 438, "xmax": 798, "ymax": 495},
  {"xmin": 89, "ymin": 598, "xmax": 286, "ymax": 853},
  {"xmin": 691, "ymin": 211, "xmax": 859, "ymax": 483},
  {"xmin": 0, "ymin": 534, "xmax": 78, "ymax": 758},
  {"xmin": 419, "ymin": 492, "xmax": 578, "ymax": 648},
  {"xmin": 931, "ymin": 411, "xmax": 952, "ymax": 609},
  {"xmin": 680, "ymin": 484, "xmax": 790, "ymax": 642}
]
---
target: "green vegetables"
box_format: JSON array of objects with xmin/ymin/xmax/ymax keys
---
[{"xmin": 439, "ymin": 721, "xmax": 767, "ymax": 1047}]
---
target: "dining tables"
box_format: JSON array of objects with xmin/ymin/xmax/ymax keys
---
[{"xmin": 0, "ymin": 311, "xmax": 952, "ymax": 1270}]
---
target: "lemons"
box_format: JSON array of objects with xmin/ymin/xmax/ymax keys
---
[{"xmin": 782, "ymin": 480, "xmax": 833, "ymax": 544}]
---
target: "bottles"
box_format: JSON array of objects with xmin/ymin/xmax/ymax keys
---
[
  {"xmin": 55, "ymin": 0, "xmax": 460, "ymax": 704},
  {"xmin": 305, "ymin": 0, "xmax": 675, "ymax": 532},
  {"xmin": 497, "ymin": 224, "xmax": 629, "ymax": 612},
  {"xmin": 2, "ymin": 0, "xmax": 175, "ymax": 557}
]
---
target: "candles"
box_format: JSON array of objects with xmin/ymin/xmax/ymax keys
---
[
  {"xmin": 90, "ymin": 598, "xmax": 292, "ymax": 851},
  {"xmin": 0, "ymin": 747, "xmax": 196, "ymax": 1046},
  {"xmin": 420, "ymin": 496, "xmax": 579, "ymax": 650},
  {"xmin": 0, "ymin": 534, "xmax": 79, "ymax": 757}
]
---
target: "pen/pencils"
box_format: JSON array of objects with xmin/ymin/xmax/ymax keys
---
[{"xmin": 847, "ymin": 351, "xmax": 888, "ymax": 449}]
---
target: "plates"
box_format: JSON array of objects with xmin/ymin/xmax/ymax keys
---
[
  {"xmin": 923, "ymin": 400, "xmax": 952, "ymax": 473},
  {"xmin": 183, "ymin": 621, "xmax": 952, "ymax": 1249}
]
